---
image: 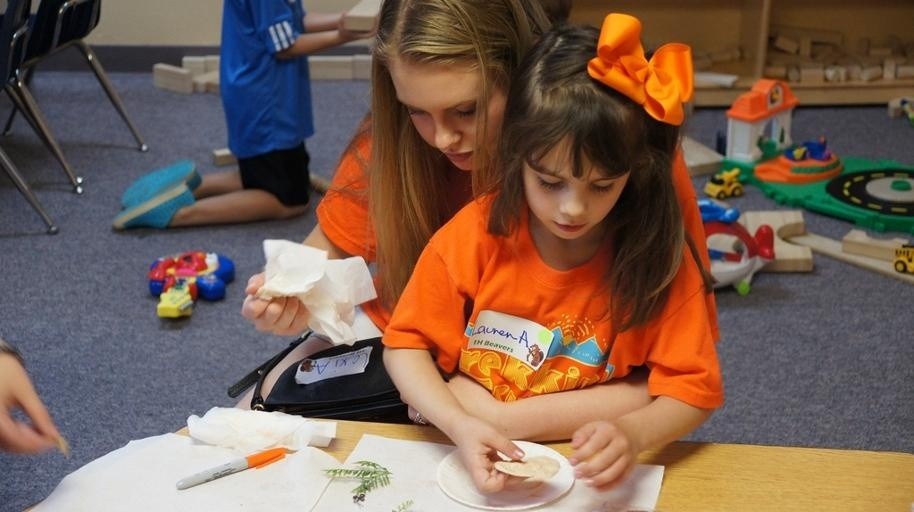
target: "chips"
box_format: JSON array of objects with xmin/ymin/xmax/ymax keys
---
[{"xmin": 494, "ymin": 456, "xmax": 561, "ymax": 480}]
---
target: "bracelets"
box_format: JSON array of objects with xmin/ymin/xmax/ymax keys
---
[{"xmin": 0, "ymin": 337, "xmax": 25, "ymax": 362}]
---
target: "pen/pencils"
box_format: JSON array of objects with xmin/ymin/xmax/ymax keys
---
[{"xmin": 176, "ymin": 447, "xmax": 288, "ymax": 490}]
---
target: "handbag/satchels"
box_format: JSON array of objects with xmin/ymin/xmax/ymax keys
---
[{"xmin": 267, "ymin": 336, "xmax": 408, "ymax": 423}]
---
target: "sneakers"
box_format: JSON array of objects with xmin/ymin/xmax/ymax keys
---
[
  {"xmin": 120, "ymin": 162, "xmax": 202, "ymax": 208},
  {"xmin": 112, "ymin": 180, "xmax": 196, "ymax": 230}
]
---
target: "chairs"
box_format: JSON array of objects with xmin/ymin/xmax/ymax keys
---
[{"xmin": 0, "ymin": 1, "xmax": 149, "ymax": 235}]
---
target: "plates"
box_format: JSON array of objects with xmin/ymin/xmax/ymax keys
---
[{"xmin": 438, "ymin": 440, "xmax": 575, "ymax": 512}]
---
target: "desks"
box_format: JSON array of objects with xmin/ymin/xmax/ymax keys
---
[{"xmin": 169, "ymin": 419, "xmax": 914, "ymax": 512}]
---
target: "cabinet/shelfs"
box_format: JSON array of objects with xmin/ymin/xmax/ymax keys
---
[{"xmin": 557, "ymin": 1, "xmax": 914, "ymax": 104}]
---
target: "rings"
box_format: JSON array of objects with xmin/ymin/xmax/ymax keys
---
[{"xmin": 413, "ymin": 412, "xmax": 426, "ymax": 425}]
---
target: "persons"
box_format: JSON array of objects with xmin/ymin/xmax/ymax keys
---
[
  {"xmin": 381, "ymin": 24, "xmax": 724, "ymax": 494},
  {"xmin": 113, "ymin": 0, "xmax": 381, "ymax": 230},
  {"xmin": 234, "ymin": 1, "xmax": 721, "ymax": 442},
  {"xmin": 0, "ymin": 339, "xmax": 60, "ymax": 454}
]
